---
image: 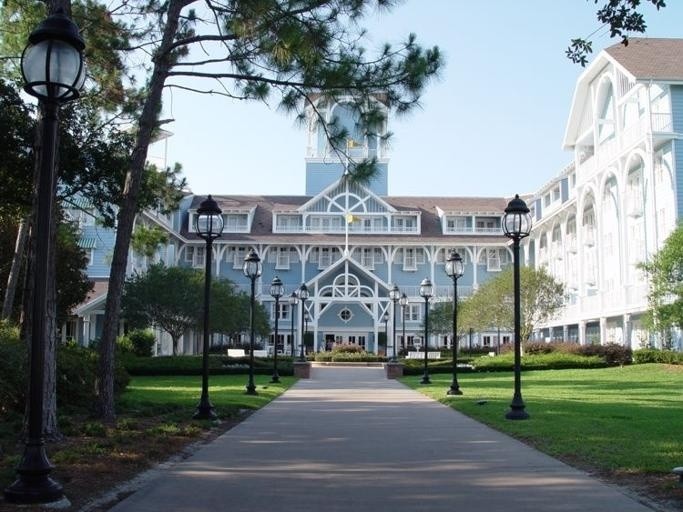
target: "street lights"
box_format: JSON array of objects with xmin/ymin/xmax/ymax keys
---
[
  {"xmin": 268, "ymin": 273, "xmax": 285, "ymax": 384},
  {"xmin": 296, "ymin": 281, "xmax": 310, "ymax": 362},
  {"xmin": 286, "ymin": 289, "xmax": 300, "ymax": 358},
  {"xmin": 418, "ymin": 277, "xmax": 435, "ymax": 386},
  {"xmin": 442, "ymin": 247, "xmax": 469, "ymax": 396},
  {"xmin": 304, "ymin": 308, "xmax": 309, "ymax": 347},
  {"xmin": 497, "ymin": 192, "xmax": 533, "ymax": 423},
  {"xmin": 382, "ymin": 309, "xmax": 390, "ymax": 356},
  {"xmin": 397, "ymin": 289, "xmax": 408, "ymax": 349},
  {"xmin": 239, "ymin": 247, "xmax": 263, "ymax": 397},
  {"xmin": 0, "ymin": 1, "xmax": 87, "ymax": 504},
  {"xmin": 387, "ymin": 281, "xmax": 400, "ymax": 363},
  {"xmin": 193, "ymin": 193, "xmax": 226, "ymax": 422}
]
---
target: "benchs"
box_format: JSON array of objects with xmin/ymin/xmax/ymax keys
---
[
  {"xmin": 249, "ymin": 349, "xmax": 269, "ymax": 358},
  {"xmin": 480, "ymin": 351, "xmax": 495, "ymax": 358},
  {"xmin": 405, "ymin": 351, "xmax": 441, "ymax": 359},
  {"xmin": 227, "ymin": 348, "xmax": 245, "ymax": 357}
]
